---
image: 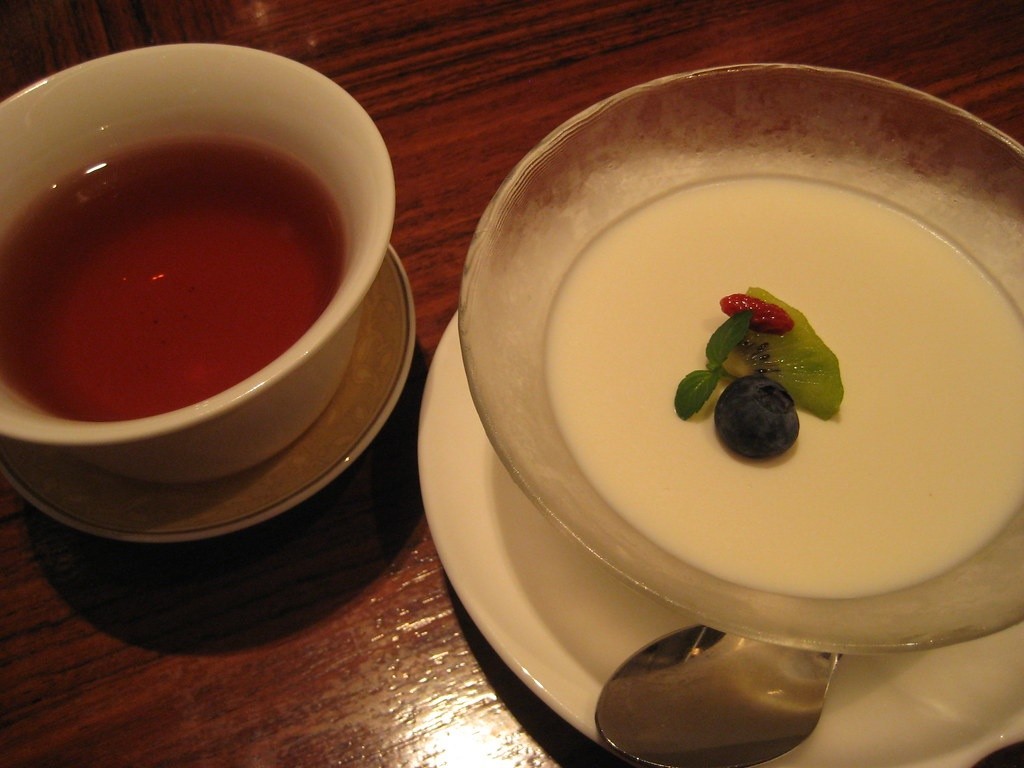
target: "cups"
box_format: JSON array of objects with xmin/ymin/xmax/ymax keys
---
[{"xmin": 3, "ymin": 42, "xmax": 393, "ymax": 482}]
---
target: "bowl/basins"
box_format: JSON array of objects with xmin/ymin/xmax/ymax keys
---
[{"xmin": 454, "ymin": 61, "xmax": 1023, "ymax": 653}]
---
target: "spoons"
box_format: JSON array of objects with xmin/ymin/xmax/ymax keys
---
[{"xmin": 595, "ymin": 627, "xmax": 839, "ymax": 765}]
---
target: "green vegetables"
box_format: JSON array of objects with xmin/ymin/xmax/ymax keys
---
[{"xmin": 675, "ymin": 309, "xmax": 754, "ymax": 420}]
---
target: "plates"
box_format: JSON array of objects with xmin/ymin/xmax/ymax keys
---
[
  {"xmin": 3, "ymin": 242, "xmax": 415, "ymax": 546},
  {"xmin": 416, "ymin": 306, "xmax": 1022, "ymax": 768}
]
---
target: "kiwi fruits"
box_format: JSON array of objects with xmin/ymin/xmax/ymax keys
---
[{"xmin": 739, "ymin": 287, "xmax": 845, "ymax": 420}]
